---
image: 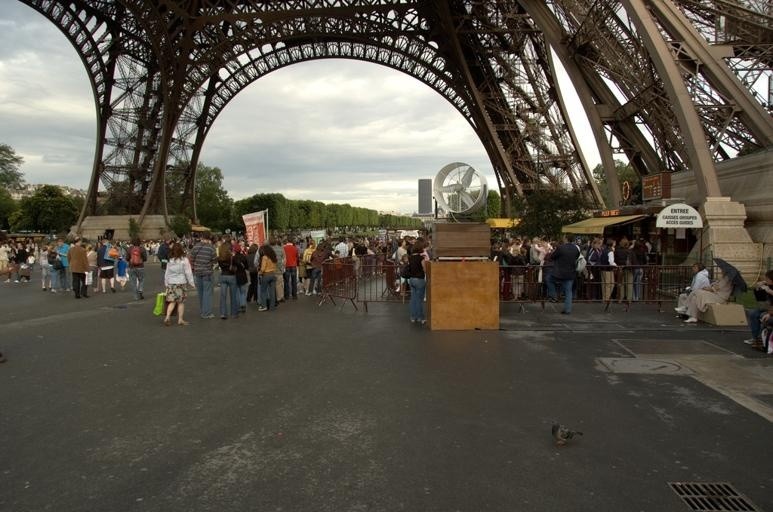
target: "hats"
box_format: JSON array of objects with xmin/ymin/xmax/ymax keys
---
[{"xmin": 233, "ymin": 245, "xmax": 241, "ymax": 252}]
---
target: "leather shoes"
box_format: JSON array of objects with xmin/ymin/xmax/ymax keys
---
[
  {"xmin": 674, "ymin": 306, "xmax": 688, "ymax": 312},
  {"xmin": 683, "ymin": 317, "xmax": 698, "ymax": 322}
]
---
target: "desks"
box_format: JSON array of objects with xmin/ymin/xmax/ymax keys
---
[{"xmin": 424, "ymin": 260, "xmax": 502, "ymax": 331}]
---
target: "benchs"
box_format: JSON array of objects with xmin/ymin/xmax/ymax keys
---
[{"xmin": 695, "ymin": 302, "xmax": 748, "ymax": 326}]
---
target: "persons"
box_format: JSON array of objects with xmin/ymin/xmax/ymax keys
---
[
  {"xmin": 751, "ymin": 311, "xmax": 773, "ymax": 355},
  {"xmin": 681, "ymin": 270, "xmax": 734, "ymax": 323},
  {"xmin": 674, "ymin": 263, "xmax": 710, "ymax": 318},
  {"xmin": 744, "ymin": 270, "xmax": 773, "ymax": 346}
]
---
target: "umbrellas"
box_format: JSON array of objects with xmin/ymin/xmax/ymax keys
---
[{"xmin": 712, "ymin": 258, "xmax": 747, "ymax": 293}]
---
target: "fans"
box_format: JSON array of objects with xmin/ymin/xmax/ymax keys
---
[{"xmin": 431, "ymin": 161, "xmax": 489, "ymax": 221}]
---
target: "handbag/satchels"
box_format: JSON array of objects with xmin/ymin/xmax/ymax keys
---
[
  {"xmin": 401, "ymin": 264, "xmax": 411, "ymax": 279},
  {"xmin": 154, "ymin": 293, "xmax": 177, "ymax": 316},
  {"xmin": 230, "ymin": 265, "xmax": 237, "ymax": 271},
  {"xmin": 108, "ymin": 248, "xmax": 119, "ymax": 258},
  {"xmin": 299, "ymin": 265, "xmax": 307, "ymax": 278},
  {"xmin": 53, "ymin": 260, "xmax": 63, "ymax": 269}
]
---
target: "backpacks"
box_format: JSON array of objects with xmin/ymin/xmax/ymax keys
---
[{"xmin": 130, "ymin": 246, "xmax": 142, "ymax": 265}]
